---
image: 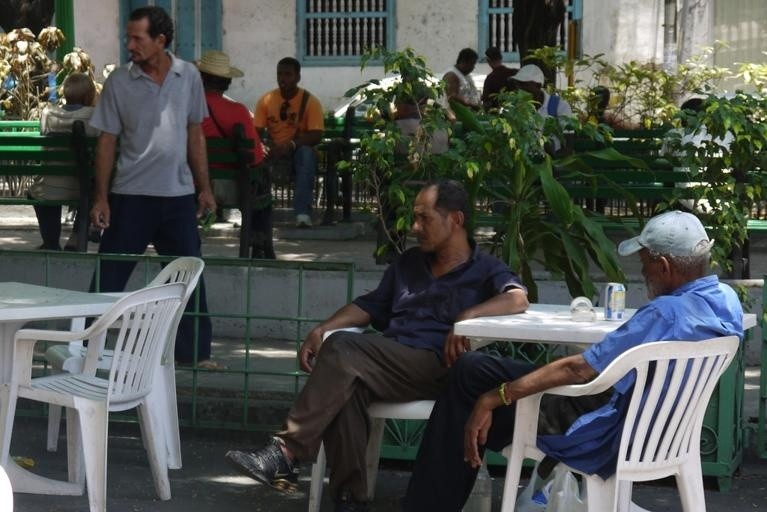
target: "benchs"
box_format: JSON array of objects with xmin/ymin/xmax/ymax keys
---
[
  {"xmin": 376, "ymin": 116, "xmax": 766, "ymax": 267},
  {"xmin": 1, "ymin": 104, "xmax": 356, "ymax": 257}
]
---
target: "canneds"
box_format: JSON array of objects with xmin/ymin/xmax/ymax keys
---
[{"xmin": 605, "ymin": 281, "xmax": 626, "ymax": 321}]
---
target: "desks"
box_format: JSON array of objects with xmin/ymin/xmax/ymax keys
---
[
  {"xmin": 453, "ymin": 303, "xmax": 756, "ymax": 512},
  {"xmin": 0, "ymin": 283, "xmax": 131, "ymax": 496}
]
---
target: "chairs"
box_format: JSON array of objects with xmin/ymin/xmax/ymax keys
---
[
  {"xmin": 47, "ymin": 255, "xmax": 204, "ymax": 490},
  {"xmin": 305, "ymin": 327, "xmax": 436, "ymax": 512},
  {"xmin": 499, "ymin": 334, "xmax": 738, "ymax": 512},
  {"xmin": 0, "ymin": 279, "xmax": 189, "ymax": 510}
]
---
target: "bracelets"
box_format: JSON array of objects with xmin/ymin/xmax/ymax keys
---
[
  {"xmin": 289, "ymin": 139, "xmax": 297, "ymax": 153},
  {"xmin": 499, "ymin": 382, "xmax": 512, "ymax": 407}
]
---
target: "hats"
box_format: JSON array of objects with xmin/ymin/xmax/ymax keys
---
[
  {"xmin": 618, "ymin": 210, "xmax": 715, "ymax": 258},
  {"xmin": 194, "ymin": 51, "xmax": 244, "ymax": 80},
  {"xmin": 510, "ymin": 65, "xmax": 544, "ymax": 87}
]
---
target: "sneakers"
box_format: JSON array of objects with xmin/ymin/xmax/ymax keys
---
[
  {"xmin": 224, "ymin": 438, "xmax": 298, "ymax": 495},
  {"xmin": 295, "ymin": 214, "xmax": 313, "ymax": 226}
]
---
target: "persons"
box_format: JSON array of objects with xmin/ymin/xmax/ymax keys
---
[
  {"xmin": 510, "ymin": 1, "xmax": 565, "ymax": 93},
  {"xmin": 76, "ymin": 5, "xmax": 222, "ymax": 377},
  {"xmin": 661, "ymin": 97, "xmax": 739, "ymax": 217},
  {"xmin": 393, "ymin": 211, "xmax": 746, "ymax": 511},
  {"xmin": 226, "ymin": 181, "xmax": 533, "ymax": 511},
  {"xmin": 580, "ymin": 85, "xmax": 620, "ymax": 129},
  {"xmin": 187, "ymin": 42, "xmax": 279, "ymax": 258},
  {"xmin": 248, "ymin": 56, "xmax": 325, "ymax": 231},
  {"xmin": 29, "ymin": 71, "xmax": 100, "ymax": 255},
  {"xmin": 392, "ymin": 47, "xmax": 574, "ymax": 171}
]
